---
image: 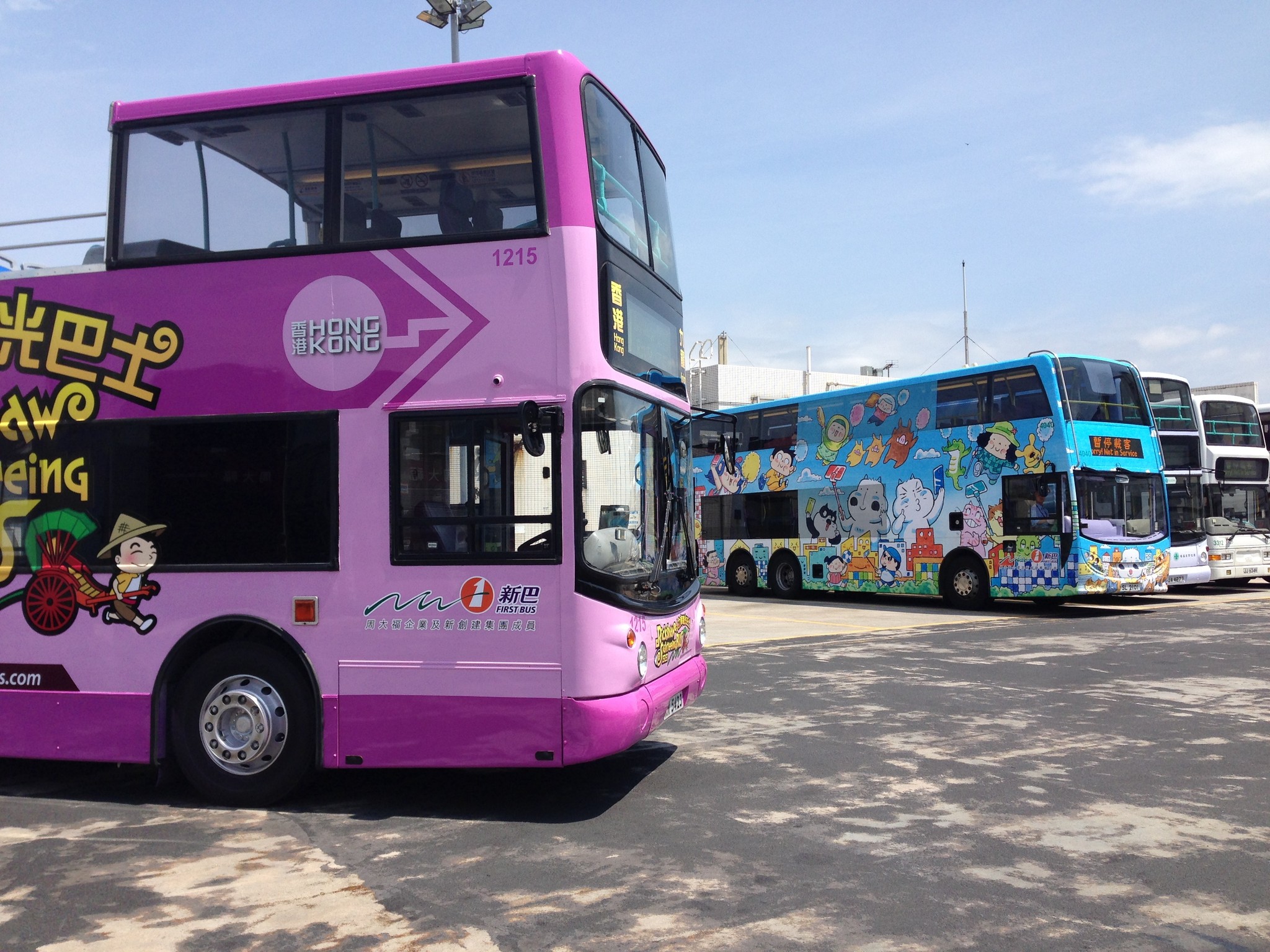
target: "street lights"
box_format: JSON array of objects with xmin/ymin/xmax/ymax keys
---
[{"xmin": 417, "ymin": 0, "xmax": 492, "ymax": 62}]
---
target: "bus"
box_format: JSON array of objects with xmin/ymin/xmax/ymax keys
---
[
  {"xmin": 0, "ymin": 49, "xmax": 706, "ymax": 804},
  {"xmin": 691, "ymin": 351, "xmax": 1270, "ymax": 612}
]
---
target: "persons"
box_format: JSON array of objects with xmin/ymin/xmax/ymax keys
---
[{"xmin": 1028, "ymin": 491, "xmax": 1055, "ymax": 533}]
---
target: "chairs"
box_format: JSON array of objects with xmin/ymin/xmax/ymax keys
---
[
  {"xmin": 371, "ymin": 210, "xmax": 401, "ymax": 239},
  {"xmin": 341, "ymin": 193, "xmax": 374, "ymax": 244},
  {"xmin": 472, "ymin": 200, "xmax": 507, "ymax": 232},
  {"xmin": 411, "ymin": 479, "xmax": 469, "ymax": 551},
  {"xmin": 439, "ymin": 179, "xmax": 474, "ymax": 235}
]
